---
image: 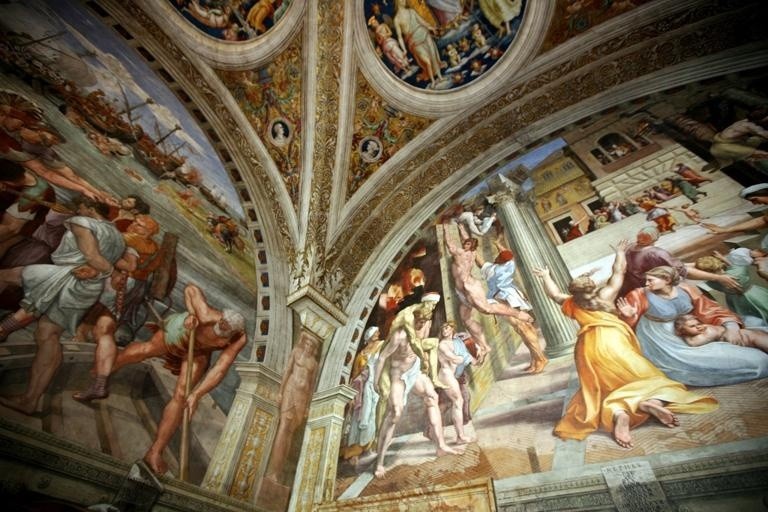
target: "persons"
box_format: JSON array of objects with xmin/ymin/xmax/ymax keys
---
[
  {"xmin": 176, "ymin": 0, "xmax": 277, "ymax": 41},
  {"xmin": 274, "ymin": 123, "xmax": 287, "ymax": 141},
  {"xmin": 368, "ymin": 0, "xmax": 522, "ymax": 90},
  {"xmin": 268, "ymin": 334, "xmax": 320, "ymax": 482}
]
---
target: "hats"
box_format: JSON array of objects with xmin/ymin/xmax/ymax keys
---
[
  {"xmin": 738, "ymin": 183, "xmax": 767, "ymax": 198},
  {"xmin": 363, "ymin": 327, "xmax": 378, "ymax": 341}
]
